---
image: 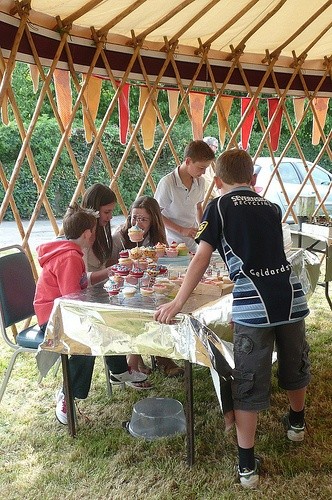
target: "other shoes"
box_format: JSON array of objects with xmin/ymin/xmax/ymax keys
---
[
  {"xmin": 155, "ymin": 355, "xmax": 184, "ymax": 379},
  {"xmin": 138, "ymin": 364, "xmax": 151, "ymax": 374},
  {"xmin": 124, "ymin": 372, "xmax": 153, "ymax": 390}
]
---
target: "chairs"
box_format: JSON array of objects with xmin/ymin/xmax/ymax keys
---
[{"xmin": 0, "ymin": 244, "xmax": 112, "ymax": 431}]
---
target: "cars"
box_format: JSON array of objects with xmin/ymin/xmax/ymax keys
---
[{"xmin": 208, "ymin": 158, "xmax": 332, "ymax": 224}]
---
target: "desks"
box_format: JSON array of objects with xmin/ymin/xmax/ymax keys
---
[
  {"xmin": 36, "ymin": 247, "xmax": 321, "ymax": 465},
  {"xmin": 289, "ymin": 222, "xmax": 332, "ymax": 311}
]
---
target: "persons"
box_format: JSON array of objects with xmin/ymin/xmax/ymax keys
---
[
  {"xmin": 201, "ymin": 137, "xmax": 221, "ymax": 196},
  {"xmin": 36, "ymin": 202, "xmax": 148, "ymax": 426},
  {"xmin": 81, "ymin": 184, "xmax": 154, "ymax": 390},
  {"xmin": 154, "ymin": 150, "xmax": 310, "ymax": 488},
  {"xmin": 107, "ymin": 196, "xmax": 184, "ymax": 376},
  {"xmin": 153, "ymin": 140, "xmax": 214, "ymax": 253}
]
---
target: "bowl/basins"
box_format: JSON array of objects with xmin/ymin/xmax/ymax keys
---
[{"xmin": 128, "ymin": 397, "xmax": 186, "ymax": 441}]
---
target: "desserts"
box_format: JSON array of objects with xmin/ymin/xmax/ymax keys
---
[
  {"xmin": 128, "ymin": 225, "xmax": 144, "ymax": 241},
  {"xmin": 200, "ymin": 275, "xmax": 233, "ymax": 289},
  {"xmin": 153, "ymin": 241, "xmax": 188, "ymax": 258},
  {"xmin": 102, "ymin": 246, "xmax": 178, "ymax": 297}
]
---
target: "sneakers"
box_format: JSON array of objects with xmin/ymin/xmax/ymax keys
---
[
  {"xmin": 286, "ymin": 419, "xmax": 305, "ymax": 442},
  {"xmin": 108, "ymin": 366, "xmax": 148, "ymax": 385},
  {"xmin": 55, "ymin": 389, "xmax": 69, "ymax": 426},
  {"xmin": 238, "ymin": 459, "xmax": 260, "ymax": 489}
]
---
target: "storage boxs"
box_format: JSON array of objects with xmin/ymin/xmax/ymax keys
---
[{"xmin": 192, "ymin": 282, "xmax": 233, "ymax": 297}]
